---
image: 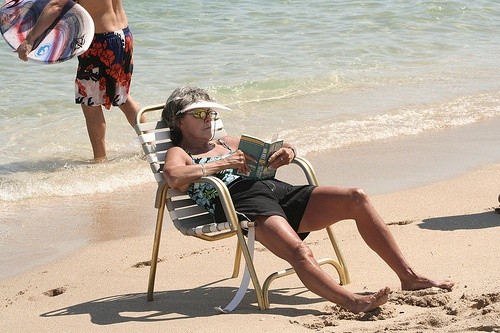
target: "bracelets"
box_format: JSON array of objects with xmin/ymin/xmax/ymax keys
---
[
  {"xmin": 200, "ymin": 162, "xmax": 205, "ymax": 177},
  {"xmin": 287, "ymin": 146, "xmax": 296, "ymax": 160}
]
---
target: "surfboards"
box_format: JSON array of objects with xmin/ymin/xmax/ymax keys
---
[{"xmin": 0, "ymin": 0, "xmax": 95, "ymax": 65}]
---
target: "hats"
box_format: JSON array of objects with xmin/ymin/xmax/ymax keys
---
[{"xmin": 157, "ymin": 83, "xmax": 233, "ymax": 128}]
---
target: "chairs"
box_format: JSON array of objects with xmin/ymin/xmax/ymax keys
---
[{"xmin": 134, "ymin": 103, "xmax": 350, "ymax": 311}]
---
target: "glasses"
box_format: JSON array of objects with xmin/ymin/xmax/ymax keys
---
[{"xmin": 179, "ymin": 110, "xmax": 218, "ymax": 119}]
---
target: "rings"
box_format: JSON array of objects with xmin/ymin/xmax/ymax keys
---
[{"xmin": 280, "ymin": 157, "xmax": 283, "ymax": 161}]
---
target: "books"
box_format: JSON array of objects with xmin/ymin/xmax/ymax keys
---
[{"xmin": 232, "ymin": 134, "xmax": 284, "ymax": 181}]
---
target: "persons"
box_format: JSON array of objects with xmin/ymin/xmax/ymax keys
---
[
  {"xmin": 160, "ymin": 85, "xmax": 456, "ymax": 314},
  {"xmin": 13, "ymin": 0, "xmax": 153, "ymax": 164}
]
